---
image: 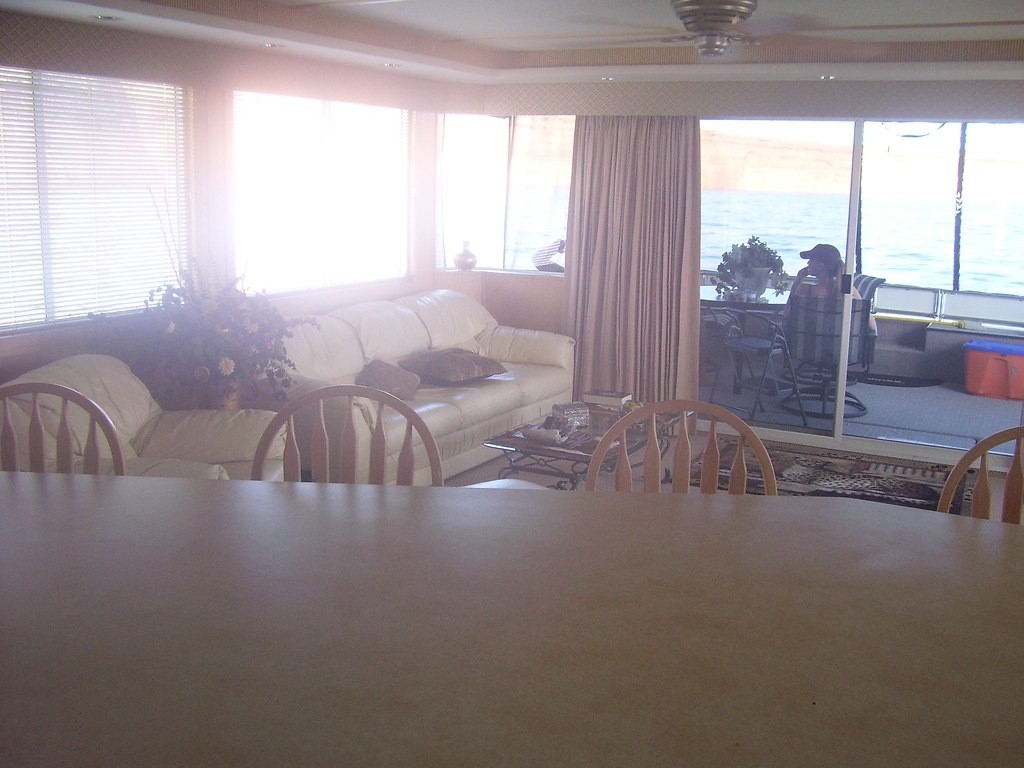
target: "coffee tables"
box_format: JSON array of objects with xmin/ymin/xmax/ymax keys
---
[{"xmin": 483, "ymin": 402, "xmax": 695, "ymax": 490}]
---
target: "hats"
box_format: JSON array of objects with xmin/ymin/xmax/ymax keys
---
[{"xmin": 800, "ymin": 244, "xmax": 841, "ymax": 261}]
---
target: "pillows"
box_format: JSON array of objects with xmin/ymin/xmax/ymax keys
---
[{"xmin": 399, "ymin": 347, "xmax": 508, "ymax": 386}]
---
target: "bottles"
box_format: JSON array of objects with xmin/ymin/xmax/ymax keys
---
[{"xmin": 454, "ymin": 240, "xmax": 477, "ymax": 271}]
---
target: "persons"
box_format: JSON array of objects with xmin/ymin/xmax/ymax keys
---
[{"xmin": 787, "ymin": 244, "xmax": 877, "ymax": 332}]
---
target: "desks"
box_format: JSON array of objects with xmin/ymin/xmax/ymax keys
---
[
  {"xmin": 0, "ymin": 471, "xmax": 1022, "ymax": 768},
  {"xmin": 699, "ymin": 285, "xmax": 792, "ymax": 396}
]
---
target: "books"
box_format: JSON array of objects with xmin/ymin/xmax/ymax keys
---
[{"xmin": 583, "ymin": 389, "xmax": 634, "ymax": 407}]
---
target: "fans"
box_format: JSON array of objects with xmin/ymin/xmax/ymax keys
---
[{"xmin": 564, "ymin": 0, "xmax": 823, "ymax": 60}]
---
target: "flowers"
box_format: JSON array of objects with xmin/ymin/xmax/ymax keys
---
[
  {"xmin": 84, "ymin": 257, "xmax": 323, "ymax": 403},
  {"xmin": 710, "ymin": 235, "xmax": 792, "ymax": 298}
]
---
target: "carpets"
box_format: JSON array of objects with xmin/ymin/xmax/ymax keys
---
[{"xmin": 689, "ymin": 438, "xmax": 979, "ymax": 517}]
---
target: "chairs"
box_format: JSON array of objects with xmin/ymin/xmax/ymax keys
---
[
  {"xmin": 0, "ymin": 383, "xmax": 123, "ymax": 476},
  {"xmin": 250, "ymin": 385, "xmax": 444, "ymax": 488},
  {"xmin": 699, "ymin": 272, "xmax": 887, "ymax": 420},
  {"xmin": 936, "ymin": 426, "xmax": 1024, "ymax": 524},
  {"xmin": 585, "ymin": 400, "xmax": 778, "ymax": 496}
]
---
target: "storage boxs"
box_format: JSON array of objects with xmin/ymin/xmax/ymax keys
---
[{"xmin": 963, "ymin": 340, "xmax": 1024, "ymax": 400}]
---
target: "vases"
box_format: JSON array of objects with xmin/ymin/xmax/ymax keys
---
[{"xmin": 736, "ymin": 267, "xmax": 773, "ymax": 296}]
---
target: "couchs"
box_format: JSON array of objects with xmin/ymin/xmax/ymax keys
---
[
  {"xmin": 0, "ymin": 354, "xmax": 287, "ymax": 482},
  {"xmin": 245, "ymin": 289, "xmax": 576, "ymax": 488}
]
